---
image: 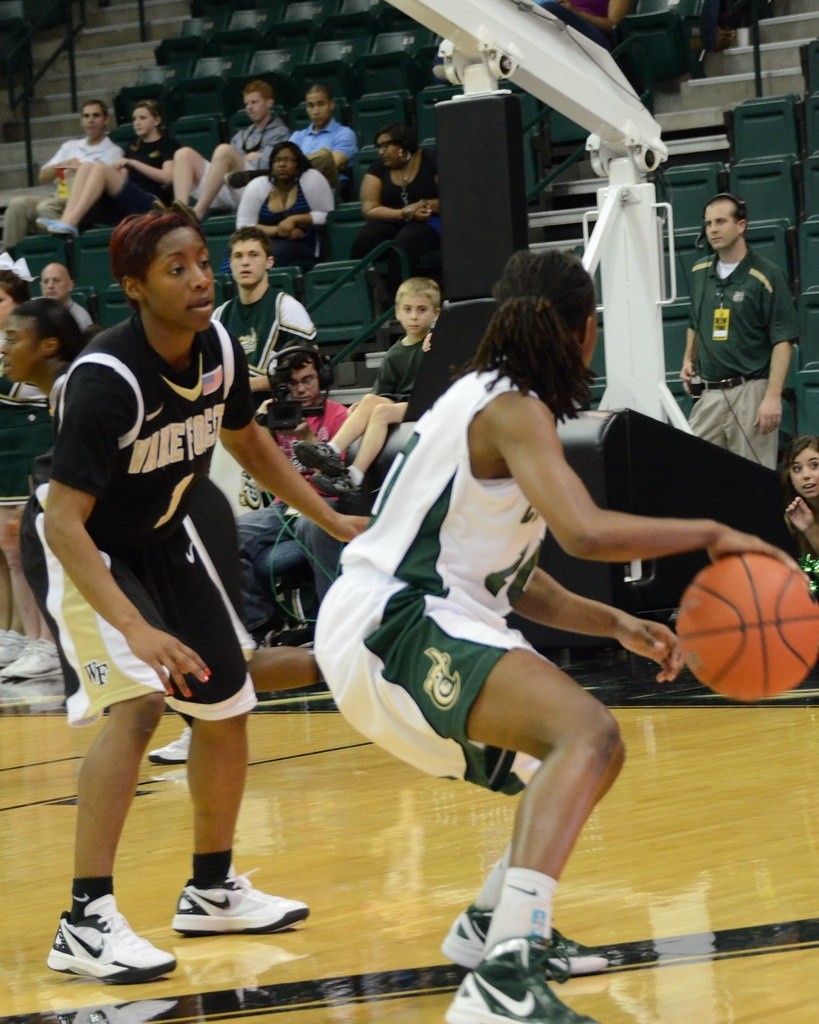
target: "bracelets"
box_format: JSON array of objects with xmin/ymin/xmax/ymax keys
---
[{"xmin": 399, "ymin": 209, "xmax": 412, "ymax": 222}]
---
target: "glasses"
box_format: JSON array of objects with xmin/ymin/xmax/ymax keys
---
[
  {"xmin": 374, "ymin": 139, "xmax": 394, "ymax": 151},
  {"xmin": 288, "ymin": 374, "xmax": 317, "ymax": 391}
]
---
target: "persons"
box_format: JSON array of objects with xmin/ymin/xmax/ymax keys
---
[
  {"xmin": 18, "ymin": 213, "xmax": 372, "ymax": 986},
  {"xmin": 780, "ymin": 434, "xmax": 819, "ymax": 560},
  {"xmin": 539, "ymin": 0, "xmax": 630, "ymax": 51},
  {"xmin": 2, "ymin": 98, "xmax": 173, "ymax": 251},
  {"xmin": 0, "ymin": 204, "xmax": 94, "ymax": 681},
  {"xmin": 234, "ymin": 340, "xmax": 349, "ymax": 648},
  {"xmin": 152, "ymin": 78, "xmax": 292, "ymax": 226},
  {"xmin": 350, "ymin": 120, "xmax": 440, "ymax": 310},
  {"xmin": 293, "ymin": 277, "xmax": 440, "ymax": 506},
  {"xmin": 680, "ymin": 195, "xmax": 804, "ymax": 471},
  {"xmin": 315, "ymin": 248, "xmax": 812, "ymax": 1024},
  {"xmin": 235, "ymin": 142, "xmax": 335, "ymax": 274},
  {"xmin": 224, "ymin": 83, "xmax": 358, "ymax": 205},
  {"xmin": 212, "ymin": 225, "xmax": 321, "ymax": 411}
]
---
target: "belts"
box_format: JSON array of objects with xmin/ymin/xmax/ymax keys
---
[{"xmin": 708, "ymin": 376, "xmax": 754, "ymax": 389}]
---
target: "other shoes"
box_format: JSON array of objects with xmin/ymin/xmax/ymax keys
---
[
  {"xmin": 224, "ymin": 169, "xmax": 254, "ymax": 189},
  {"xmin": 36, "ymin": 218, "xmax": 79, "ymax": 238},
  {"xmin": 153, "ymin": 199, "xmax": 169, "ymax": 211},
  {"xmin": 173, "ymin": 200, "xmax": 202, "ymax": 232}
]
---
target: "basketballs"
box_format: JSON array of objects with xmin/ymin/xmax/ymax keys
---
[{"xmin": 676, "ymin": 550, "xmax": 819, "ymax": 703}]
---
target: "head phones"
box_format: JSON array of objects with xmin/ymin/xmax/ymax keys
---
[
  {"xmin": 266, "ymin": 346, "xmax": 334, "ymax": 397},
  {"xmin": 701, "ymin": 193, "xmax": 747, "ymax": 238}
]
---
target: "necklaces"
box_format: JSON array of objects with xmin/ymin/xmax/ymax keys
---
[{"xmin": 399, "ymin": 153, "xmax": 415, "ymax": 205}]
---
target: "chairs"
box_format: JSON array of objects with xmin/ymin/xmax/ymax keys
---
[{"xmin": 1, "ymin": 1, "xmax": 818, "ymax": 459}]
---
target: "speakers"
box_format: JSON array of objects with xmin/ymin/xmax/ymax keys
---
[{"xmin": 431, "ymin": 95, "xmax": 529, "ymax": 305}]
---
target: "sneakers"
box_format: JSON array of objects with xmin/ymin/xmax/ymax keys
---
[
  {"xmin": 174, "ymin": 938, "xmax": 308, "ymax": 985},
  {"xmin": 171, "ymin": 866, "xmax": 309, "ymax": 933},
  {"xmin": 444, "ymin": 937, "xmax": 600, "ymax": 1024},
  {"xmin": 47, "ymin": 894, "xmax": 178, "ymax": 985},
  {"xmin": 440, "ymin": 905, "xmax": 608, "ymax": 977},
  {"xmin": 55, "ymin": 999, "xmax": 178, "ymax": 1024},
  {"xmin": 0, "ymin": 642, "xmax": 34, "ymax": 679},
  {"xmin": 0, "ymin": 629, "xmax": 31, "ymax": 667},
  {"xmin": 11, "ymin": 639, "xmax": 64, "ymax": 678},
  {"xmin": 295, "ymin": 441, "xmax": 341, "ymax": 476},
  {"xmin": 0, "ymin": 678, "xmax": 65, "ymax": 711},
  {"xmin": 312, "ymin": 469, "xmax": 363, "ymax": 503},
  {"xmin": 147, "ymin": 727, "xmax": 193, "ymax": 764}
]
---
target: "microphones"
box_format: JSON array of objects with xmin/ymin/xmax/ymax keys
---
[{"xmin": 695, "ymin": 232, "xmax": 704, "ymax": 250}]
